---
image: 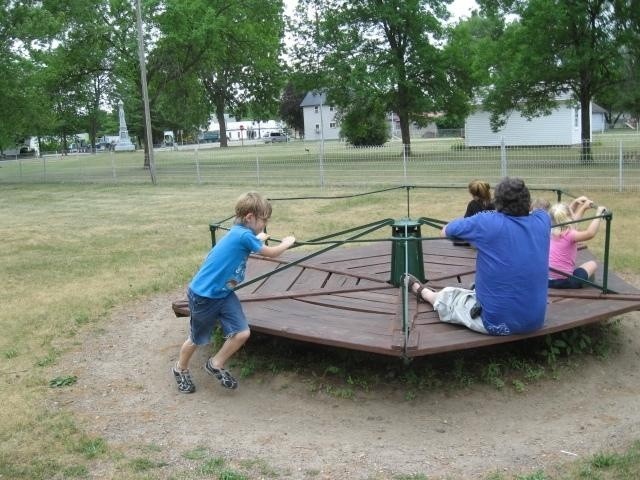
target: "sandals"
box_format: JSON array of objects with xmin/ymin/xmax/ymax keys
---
[{"xmin": 400, "ymin": 273, "xmax": 436, "ymax": 303}]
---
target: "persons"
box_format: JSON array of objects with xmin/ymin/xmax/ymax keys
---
[
  {"xmin": 462, "ymin": 180, "xmax": 496, "ymax": 218},
  {"xmin": 170, "ymin": 190, "xmax": 295, "ymax": 395},
  {"xmin": 547, "ymin": 200, "xmax": 607, "ymax": 290},
  {"xmin": 399, "ymin": 178, "xmax": 552, "ymax": 335},
  {"xmin": 531, "ymin": 193, "xmax": 588, "ymax": 220}
]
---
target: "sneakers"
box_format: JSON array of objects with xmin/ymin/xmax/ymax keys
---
[
  {"xmin": 204, "ymin": 357, "xmax": 238, "ymax": 390},
  {"xmin": 172, "ymin": 361, "xmax": 195, "ymax": 393}
]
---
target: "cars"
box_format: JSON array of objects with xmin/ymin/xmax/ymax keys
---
[{"xmin": 87, "ymin": 141, "xmax": 113, "ymax": 150}]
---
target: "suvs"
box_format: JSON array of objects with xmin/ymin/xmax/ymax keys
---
[{"xmin": 262, "ymin": 131, "xmax": 291, "ymax": 143}]
